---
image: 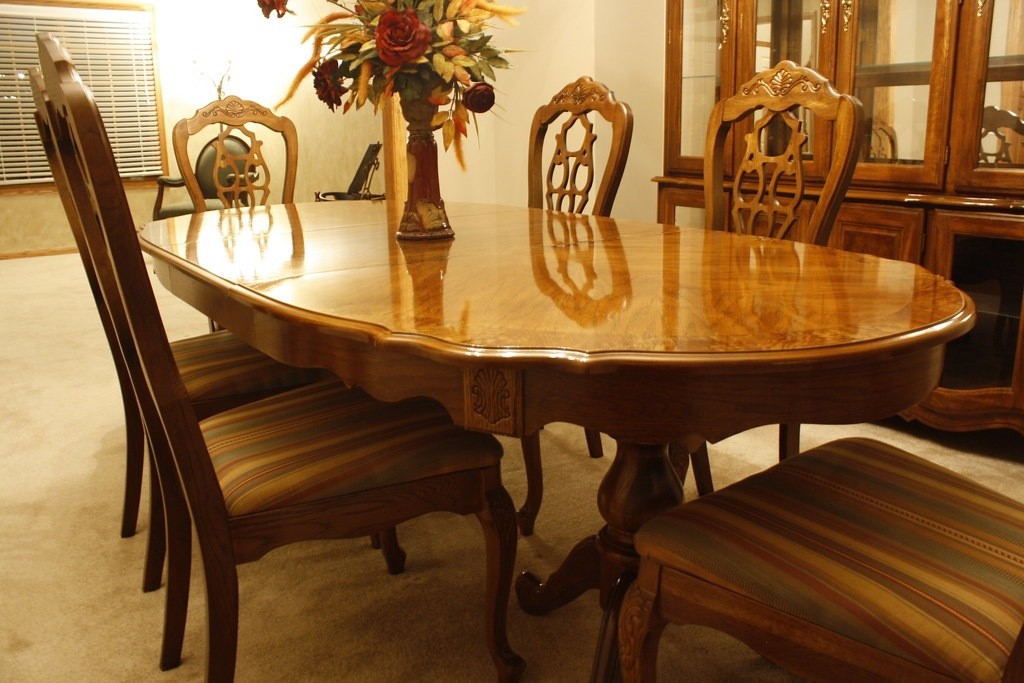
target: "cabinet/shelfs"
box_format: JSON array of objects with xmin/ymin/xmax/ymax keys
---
[{"xmin": 651, "ymin": 1, "xmax": 1023, "ymax": 438}]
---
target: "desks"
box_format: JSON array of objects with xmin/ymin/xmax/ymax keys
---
[{"xmin": 136, "ymin": 200, "xmax": 973, "ymax": 682}]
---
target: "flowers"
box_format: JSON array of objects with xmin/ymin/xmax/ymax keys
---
[{"xmin": 254, "ymin": 0, "xmax": 528, "ymax": 166}]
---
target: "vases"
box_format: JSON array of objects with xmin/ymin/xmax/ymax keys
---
[{"xmin": 381, "ymin": 66, "xmax": 455, "ymax": 238}]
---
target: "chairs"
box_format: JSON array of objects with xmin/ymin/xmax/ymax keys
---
[
  {"xmin": 28, "ymin": 68, "xmax": 408, "ymax": 595},
  {"xmin": 527, "ymin": 75, "xmax": 632, "ymax": 460},
  {"xmin": 171, "ymin": 95, "xmax": 299, "ymax": 332},
  {"xmin": 28, "ymin": 33, "xmax": 530, "ymax": 683},
  {"xmin": 668, "ymin": 58, "xmax": 874, "ymax": 494},
  {"xmin": 617, "ymin": 446, "xmax": 1023, "ymax": 682},
  {"xmin": 152, "ymin": 137, "xmax": 260, "ymax": 220}
]
